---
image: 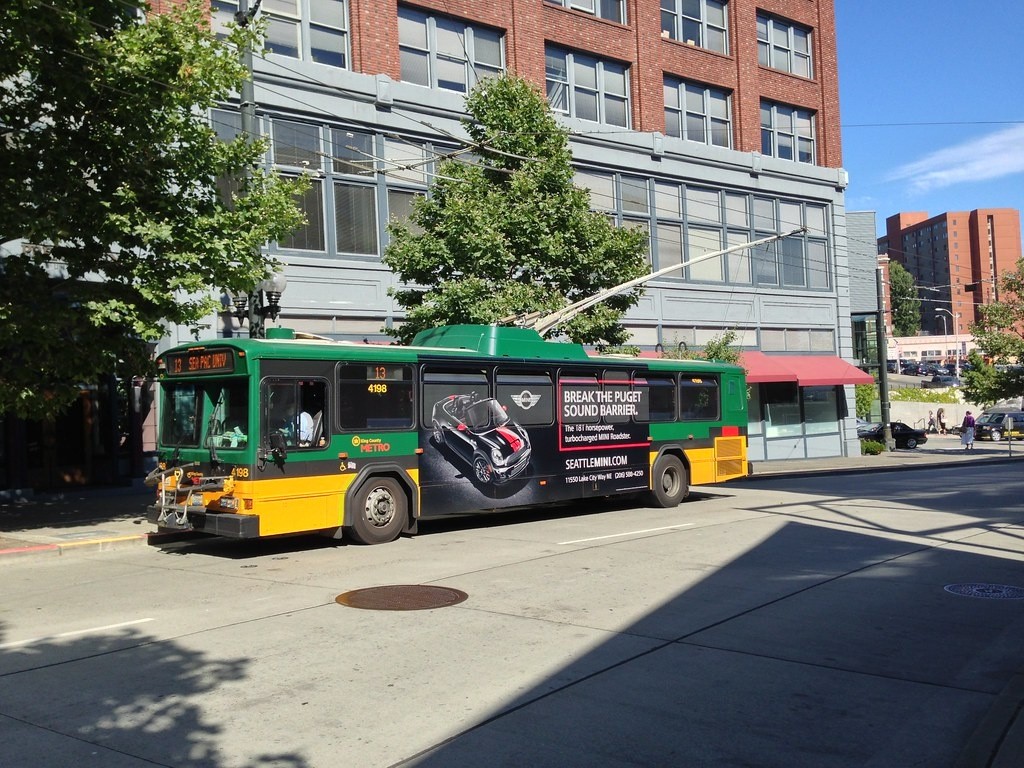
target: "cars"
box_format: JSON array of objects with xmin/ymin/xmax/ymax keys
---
[
  {"xmin": 430, "ymin": 391, "xmax": 533, "ymax": 488},
  {"xmin": 857, "ymin": 422, "xmax": 928, "ymax": 450},
  {"xmin": 974, "ymin": 406, "xmax": 1021, "ymax": 424},
  {"xmin": 975, "ymin": 412, "xmax": 1024, "ymax": 441},
  {"xmin": 884, "ymin": 358, "xmax": 1024, "ymax": 377}
]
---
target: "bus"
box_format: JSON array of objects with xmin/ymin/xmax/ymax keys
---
[{"xmin": 149, "ymin": 320, "xmax": 751, "ymax": 549}]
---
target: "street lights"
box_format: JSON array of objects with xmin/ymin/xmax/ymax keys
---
[
  {"xmin": 935, "ymin": 307, "xmax": 960, "ymax": 379},
  {"xmin": 935, "ymin": 314, "xmax": 948, "ymax": 365}
]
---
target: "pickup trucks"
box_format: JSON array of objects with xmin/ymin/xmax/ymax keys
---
[{"xmin": 921, "ymin": 375, "xmax": 963, "ymax": 388}]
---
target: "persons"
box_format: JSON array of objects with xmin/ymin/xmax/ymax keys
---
[
  {"xmin": 937, "ymin": 409, "xmax": 947, "ymax": 435},
  {"xmin": 959, "ymin": 410, "xmax": 976, "ymax": 449},
  {"xmin": 283, "ymin": 395, "xmax": 314, "ymax": 448},
  {"xmin": 927, "ymin": 410, "xmax": 939, "ymax": 435}
]
